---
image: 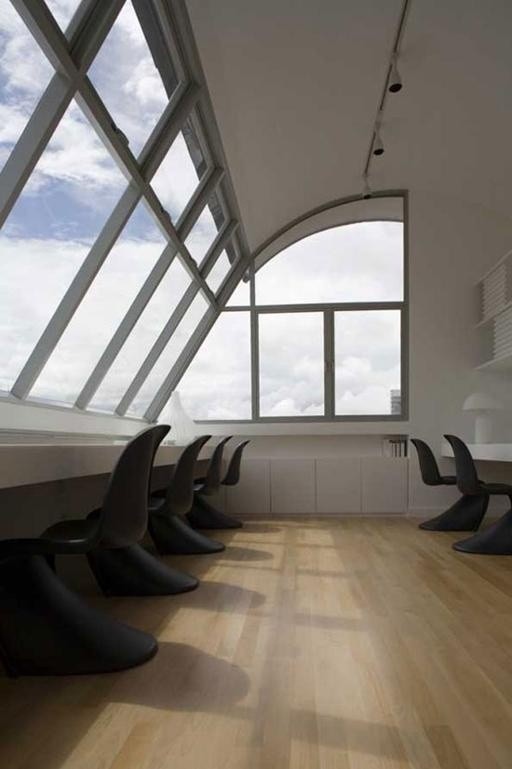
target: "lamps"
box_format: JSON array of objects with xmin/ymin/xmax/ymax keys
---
[
  {"xmin": 461, "ymin": 390, "xmax": 505, "ymax": 443},
  {"xmin": 360, "ymin": 54, "xmax": 403, "ymax": 202}
]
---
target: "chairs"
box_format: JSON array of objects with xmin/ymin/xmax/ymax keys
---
[
  {"xmin": 85, "ymin": 435, "xmax": 199, "ymax": 599},
  {"xmin": 1, "ymin": 424, "xmax": 172, "ymax": 676},
  {"xmin": 193, "ymin": 440, "xmax": 250, "ymax": 529},
  {"xmin": 146, "ymin": 437, "xmax": 235, "ymax": 556},
  {"xmin": 409, "ymin": 434, "xmax": 512, "ymax": 556}
]
